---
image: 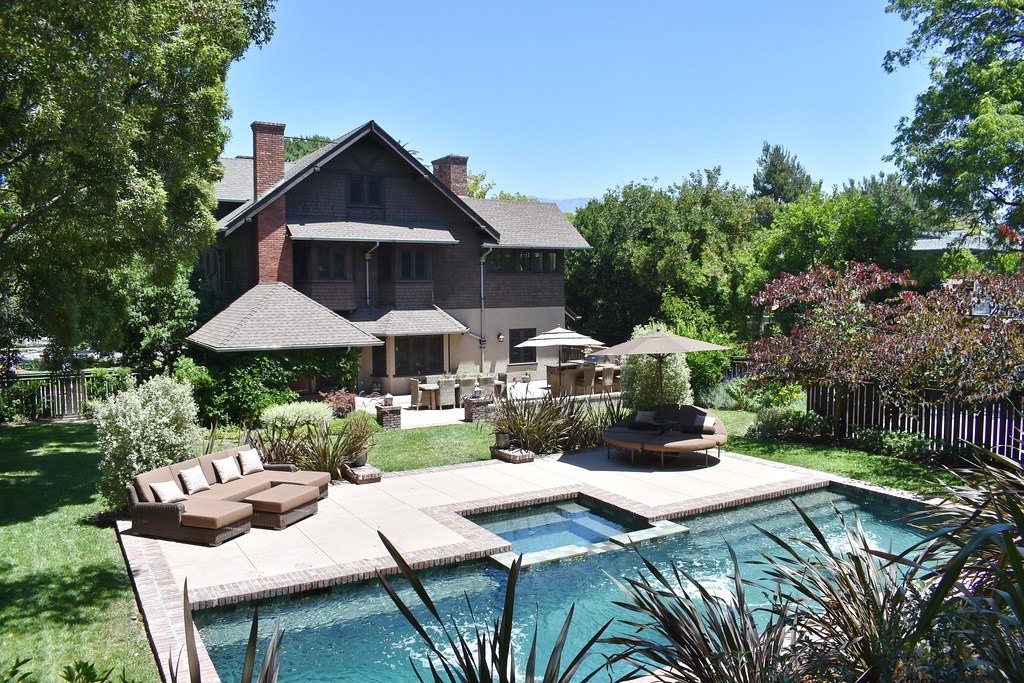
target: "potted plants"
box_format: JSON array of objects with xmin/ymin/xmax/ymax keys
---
[
  {"xmin": 344, "ymin": 419, "xmax": 374, "ymax": 466},
  {"xmin": 494, "ymin": 414, "xmax": 511, "ymax": 447},
  {"xmin": 522, "ymin": 370, "xmax": 531, "ymax": 382}
]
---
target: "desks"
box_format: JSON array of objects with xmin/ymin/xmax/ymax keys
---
[
  {"xmin": 418, "ymin": 380, "xmax": 505, "ymax": 410},
  {"xmin": 550, "ymin": 368, "xmax": 621, "ymax": 397}
]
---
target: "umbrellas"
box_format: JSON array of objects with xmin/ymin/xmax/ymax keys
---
[
  {"xmin": 514, "ymin": 324, "xmax": 605, "ymax": 395},
  {"xmin": 590, "ymin": 328, "xmax": 732, "ymax": 429}
]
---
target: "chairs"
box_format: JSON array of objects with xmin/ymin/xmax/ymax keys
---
[
  {"xmin": 410, "ymin": 378, "xmax": 431, "ymax": 411},
  {"xmin": 598, "ymin": 368, "xmax": 614, "ymax": 393},
  {"xmin": 477, "ymin": 377, "xmax": 495, "ymax": 397},
  {"xmin": 436, "ymin": 379, "xmax": 456, "ymax": 410},
  {"xmin": 458, "ymin": 378, "xmax": 476, "ymax": 409},
  {"xmin": 583, "ymin": 368, "xmax": 594, "ymax": 394},
  {"xmin": 498, "ymin": 373, "xmax": 508, "ymax": 400}
]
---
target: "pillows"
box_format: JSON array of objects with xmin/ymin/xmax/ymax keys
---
[
  {"xmin": 681, "ymin": 424, "xmax": 715, "ymax": 435},
  {"xmin": 694, "ymin": 414, "xmax": 717, "ymax": 426},
  {"xmin": 237, "ymin": 448, "xmax": 264, "ymax": 475},
  {"xmin": 149, "ymin": 480, "xmax": 188, "ymax": 503},
  {"xmin": 212, "ymin": 456, "xmax": 242, "ymax": 484},
  {"xmin": 635, "ymin": 410, "xmax": 656, "ymax": 423},
  {"xmin": 179, "ymin": 465, "xmax": 211, "ymax": 496},
  {"xmin": 628, "ymin": 422, "xmax": 658, "ymax": 430}
]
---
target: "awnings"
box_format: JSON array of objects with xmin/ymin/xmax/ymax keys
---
[{"xmin": 287, "ymin": 215, "xmax": 459, "ymax": 245}]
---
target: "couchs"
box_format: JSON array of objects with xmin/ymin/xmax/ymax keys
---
[{"xmin": 125, "ymin": 443, "xmax": 331, "ymax": 544}]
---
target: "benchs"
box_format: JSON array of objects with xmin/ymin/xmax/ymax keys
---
[
  {"xmin": 243, "ymin": 484, "xmax": 319, "ymax": 529},
  {"xmin": 604, "ymin": 405, "xmax": 727, "ymax": 467}
]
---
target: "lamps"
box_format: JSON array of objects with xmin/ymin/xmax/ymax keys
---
[
  {"xmin": 472, "ymin": 387, "xmax": 481, "ymax": 397},
  {"xmin": 498, "ymin": 335, "xmax": 505, "ymax": 343},
  {"xmin": 383, "ymin": 393, "xmax": 393, "ymax": 406}
]
